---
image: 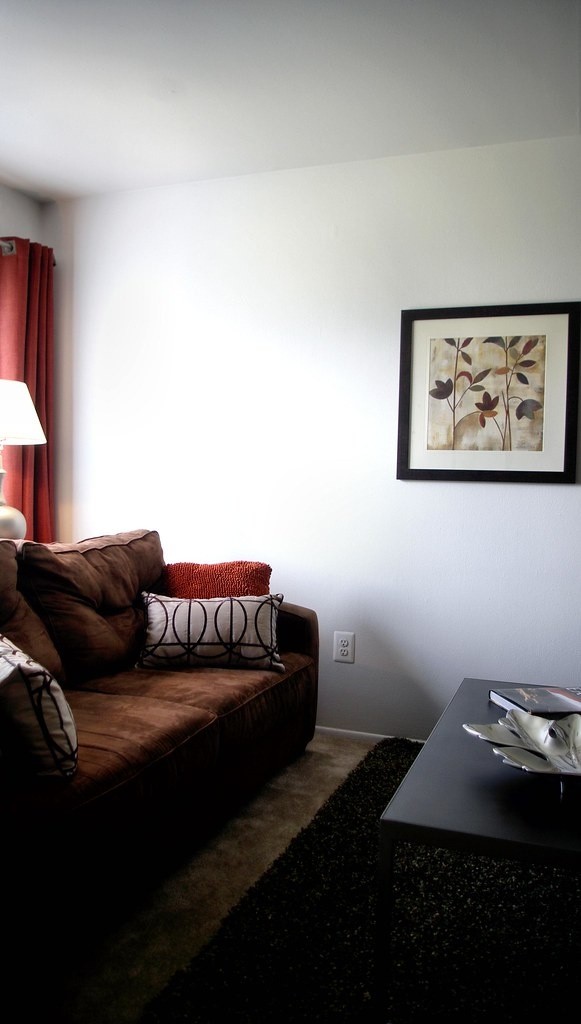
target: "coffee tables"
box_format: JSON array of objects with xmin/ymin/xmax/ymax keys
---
[{"xmin": 370, "ymin": 678, "xmax": 581, "ymax": 977}]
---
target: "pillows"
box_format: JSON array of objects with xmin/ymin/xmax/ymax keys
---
[
  {"xmin": 1, "ymin": 632, "xmax": 80, "ymax": 778},
  {"xmin": 135, "ymin": 590, "xmax": 287, "ymax": 676},
  {"xmin": 162, "ymin": 561, "xmax": 273, "ymax": 598}
]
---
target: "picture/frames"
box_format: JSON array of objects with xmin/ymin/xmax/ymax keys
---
[{"xmin": 394, "ymin": 301, "xmax": 581, "ymax": 485}]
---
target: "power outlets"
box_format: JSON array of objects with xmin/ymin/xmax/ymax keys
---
[{"xmin": 332, "ymin": 630, "xmax": 355, "ymax": 664}]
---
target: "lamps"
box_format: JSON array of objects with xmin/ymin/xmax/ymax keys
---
[{"xmin": 0, "ymin": 378, "xmax": 48, "ymax": 539}]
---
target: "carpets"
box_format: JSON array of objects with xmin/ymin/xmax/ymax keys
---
[{"xmin": 142, "ymin": 734, "xmax": 580, "ymax": 1024}]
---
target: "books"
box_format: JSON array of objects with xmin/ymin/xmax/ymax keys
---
[{"xmin": 488, "ymin": 687, "xmax": 581, "ymax": 720}]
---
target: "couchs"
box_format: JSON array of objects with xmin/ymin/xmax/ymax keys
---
[{"xmin": 0, "ymin": 597, "xmax": 319, "ymax": 956}]
